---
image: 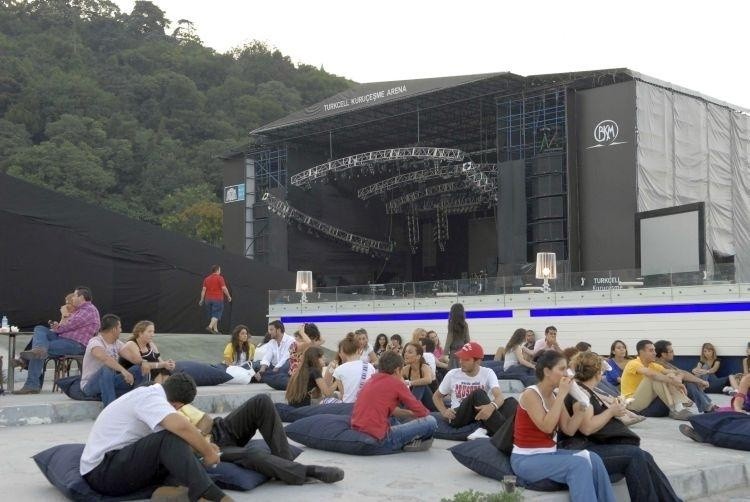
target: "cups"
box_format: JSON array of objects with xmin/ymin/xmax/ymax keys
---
[{"xmin": 502, "ymin": 474, "xmax": 517, "ymax": 494}]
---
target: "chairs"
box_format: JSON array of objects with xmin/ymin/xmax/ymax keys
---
[{"xmin": 50, "ymin": 326, "xmax": 100, "ymax": 394}]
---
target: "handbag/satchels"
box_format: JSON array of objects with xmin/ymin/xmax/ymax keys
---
[{"xmin": 490, "ymin": 414, "xmax": 516, "ymax": 455}]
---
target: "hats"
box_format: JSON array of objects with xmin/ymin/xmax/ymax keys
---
[{"xmin": 454, "ymin": 342, "xmax": 484, "ymax": 359}]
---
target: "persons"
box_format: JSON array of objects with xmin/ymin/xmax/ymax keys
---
[
  {"xmin": 507, "ymin": 349, "xmax": 619, "ymax": 501},
  {"xmin": 77, "ymin": 372, "xmax": 235, "ymax": 502},
  {"xmin": 197, "ymin": 264, "xmax": 233, "ymax": 334},
  {"xmin": 557, "ymin": 350, "xmax": 685, "ymax": 501},
  {"xmin": 222, "ymin": 303, "xmax": 518, "ymax": 454},
  {"xmin": 148, "ymin": 369, "xmax": 346, "ymax": 486},
  {"xmin": 10, "ymin": 286, "xmax": 176, "ymax": 410},
  {"xmin": 494, "ymin": 326, "xmax": 750, "ymax": 444}
]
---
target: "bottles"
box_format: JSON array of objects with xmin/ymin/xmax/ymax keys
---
[{"xmin": 2, "ymin": 315, "xmax": 9, "ymax": 327}]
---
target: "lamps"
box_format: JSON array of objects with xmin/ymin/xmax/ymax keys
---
[
  {"xmin": 294, "ymin": 268, "xmax": 315, "ymax": 304},
  {"xmin": 533, "ymin": 249, "xmax": 559, "ymax": 292}
]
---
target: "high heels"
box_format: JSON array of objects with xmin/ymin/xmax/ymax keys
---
[{"xmin": 8, "ymin": 358, "xmax": 26, "ymax": 372}]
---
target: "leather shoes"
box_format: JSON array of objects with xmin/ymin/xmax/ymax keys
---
[
  {"xmin": 214, "ymin": 331, "xmax": 222, "ymax": 335},
  {"xmin": 197, "ymin": 494, "xmax": 237, "ymax": 502},
  {"xmin": 151, "ymin": 486, "xmax": 191, "ymax": 501},
  {"xmin": 11, "ymin": 387, "xmax": 40, "ymax": 394},
  {"xmin": 313, "ymin": 466, "xmax": 344, "ymax": 484},
  {"xmin": 20, "ymin": 348, "xmax": 48, "ymax": 360},
  {"xmin": 205, "ymin": 326, "xmax": 216, "ymax": 334}
]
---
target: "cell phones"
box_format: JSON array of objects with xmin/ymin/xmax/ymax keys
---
[{"xmin": 48, "ymin": 320, "xmax": 60, "ymax": 329}]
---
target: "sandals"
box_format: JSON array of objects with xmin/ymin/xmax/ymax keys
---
[
  {"xmin": 620, "ymin": 416, "xmax": 639, "ymax": 427},
  {"xmin": 629, "ymin": 411, "xmax": 647, "ymax": 423}
]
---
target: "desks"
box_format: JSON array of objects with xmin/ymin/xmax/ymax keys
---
[{"xmin": 0, "ymin": 330, "xmax": 40, "ymax": 396}]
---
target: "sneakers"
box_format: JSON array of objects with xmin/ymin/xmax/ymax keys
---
[
  {"xmin": 669, "ymin": 387, "xmax": 740, "ymax": 444},
  {"xmin": 402, "ymin": 434, "xmax": 433, "ymax": 453},
  {"xmin": 466, "ymin": 433, "xmax": 491, "ymax": 440}
]
